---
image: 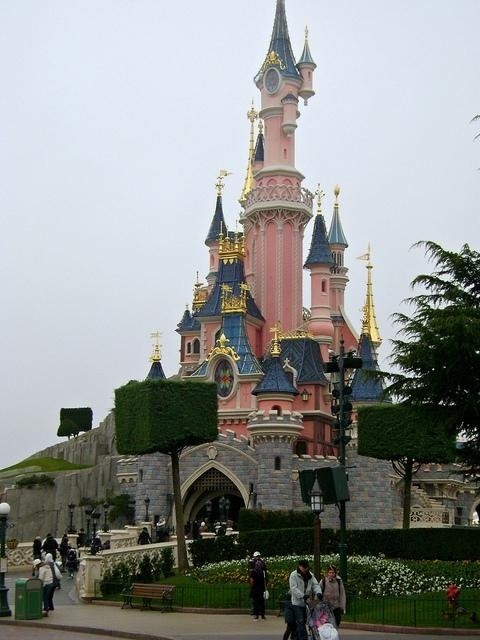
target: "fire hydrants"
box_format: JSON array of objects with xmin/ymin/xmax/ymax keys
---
[{"xmin": 446, "ymin": 583, "xmax": 459, "ymax": 608}]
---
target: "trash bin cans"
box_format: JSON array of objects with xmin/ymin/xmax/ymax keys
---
[
  {"xmin": 14, "ymin": 578, "xmax": 43, "ymax": 620},
  {"xmin": 156, "ymin": 527, "xmax": 170, "ymax": 543}
]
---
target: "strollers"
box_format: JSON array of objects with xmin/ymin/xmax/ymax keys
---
[{"xmin": 306, "ymin": 598, "xmax": 341, "ymax": 640}]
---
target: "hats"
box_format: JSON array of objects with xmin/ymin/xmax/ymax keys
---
[
  {"xmin": 298, "ymin": 560, "xmax": 309, "ymax": 569},
  {"xmin": 253, "ymin": 551, "xmax": 261, "ymax": 556},
  {"xmin": 33, "ymin": 559, "xmax": 41, "ymax": 568}
]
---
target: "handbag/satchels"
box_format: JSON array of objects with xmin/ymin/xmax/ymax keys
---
[{"xmin": 263, "ymin": 590, "xmax": 269, "ymax": 600}]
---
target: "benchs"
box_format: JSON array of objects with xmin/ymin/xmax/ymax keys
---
[{"xmin": 120, "ymin": 582, "xmax": 176, "ymax": 613}]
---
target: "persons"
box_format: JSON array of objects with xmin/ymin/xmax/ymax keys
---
[
  {"xmin": 33, "ymin": 529, "xmax": 89, "ymax": 610},
  {"xmin": 249, "ymin": 561, "xmax": 268, "ymax": 622},
  {"xmin": 155, "ymin": 525, "xmax": 171, "ymax": 542},
  {"xmin": 313, "ymin": 565, "xmax": 346, "ymax": 629},
  {"xmin": 283, "ymin": 593, "xmax": 297, "ymax": 640},
  {"xmin": 248, "ymin": 551, "xmax": 268, "ymax": 615},
  {"xmin": 137, "ymin": 527, "xmax": 153, "ymax": 545},
  {"xmin": 290, "ymin": 561, "xmax": 322, "ymax": 640}
]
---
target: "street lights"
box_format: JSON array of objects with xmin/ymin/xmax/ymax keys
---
[
  {"xmin": 84, "ymin": 503, "xmax": 101, "ymax": 555},
  {"xmin": 0, "ymin": 502, "xmax": 11, "ymax": 616},
  {"xmin": 143, "ymin": 493, "xmax": 150, "ymax": 522},
  {"xmin": 309, "ymin": 471, "xmax": 324, "ymax": 582},
  {"xmin": 68, "ymin": 500, "xmax": 76, "ymax": 535},
  {"xmin": 103, "ymin": 496, "xmax": 110, "ymax": 533}
]
